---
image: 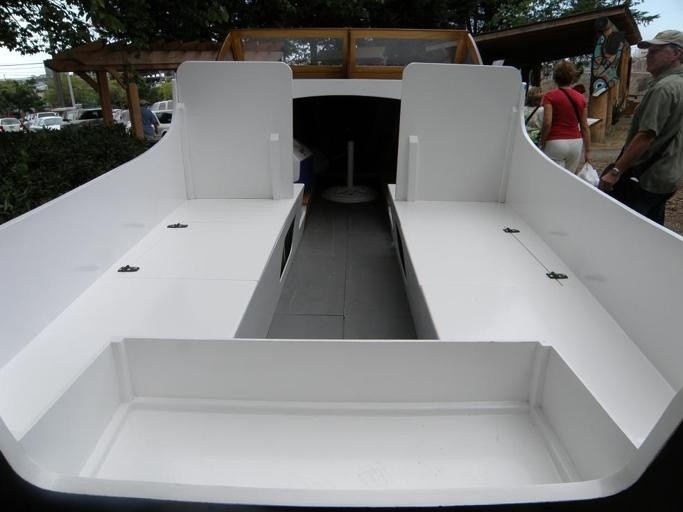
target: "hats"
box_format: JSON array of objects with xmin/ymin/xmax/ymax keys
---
[{"xmin": 637, "ymin": 29, "xmax": 683, "ymax": 49}]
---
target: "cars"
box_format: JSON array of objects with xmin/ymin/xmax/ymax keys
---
[{"xmin": 0, "ymin": 99, "xmax": 176, "ymax": 142}]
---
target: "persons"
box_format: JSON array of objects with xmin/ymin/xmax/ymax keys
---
[
  {"xmin": 138, "ymin": 100, "xmax": 160, "ymax": 140},
  {"xmin": 119, "ymin": 105, "xmax": 128, "ymax": 128},
  {"xmin": 540, "ymin": 60, "xmax": 593, "ymax": 175},
  {"xmin": 598, "ymin": 29, "xmax": 682, "ymax": 229},
  {"xmin": 521, "ymin": 86, "xmax": 545, "ymax": 149}
]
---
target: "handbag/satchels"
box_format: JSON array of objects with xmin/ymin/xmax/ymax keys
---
[{"xmin": 600, "ymin": 164, "xmax": 641, "ymax": 200}]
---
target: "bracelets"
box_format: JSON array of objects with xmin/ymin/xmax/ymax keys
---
[{"xmin": 612, "ymin": 167, "xmax": 620, "ymax": 176}]
